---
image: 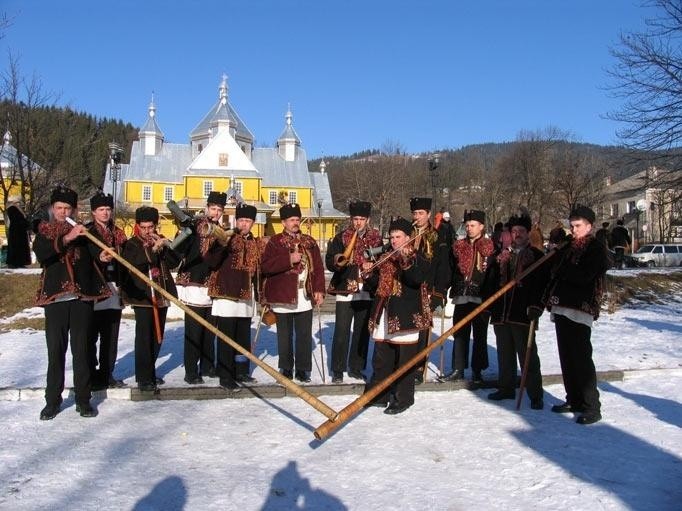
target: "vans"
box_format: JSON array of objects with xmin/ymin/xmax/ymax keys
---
[{"xmin": 630, "ymin": 244, "xmax": 682, "ymax": 267}]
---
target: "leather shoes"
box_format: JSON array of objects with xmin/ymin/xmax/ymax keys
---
[
  {"xmin": 364, "ymin": 400, "xmax": 387, "ymax": 406},
  {"xmin": 473, "ymin": 378, "xmax": 490, "ymax": 387},
  {"xmin": 235, "ymin": 375, "xmax": 257, "ymax": 384},
  {"xmin": 76, "ymin": 404, "xmax": 97, "ymax": 416},
  {"xmin": 488, "ymin": 390, "xmax": 515, "ymax": 400},
  {"xmin": 141, "ymin": 384, "xmax": 159, "ymax": 392},
  {"xmin": 295, "ymin": 375, "xmax": 311, "ymax": 382},
  {"xmin": 384, "ymin": 400, "xmax": 414, "ymax": 414},
  {"xmin": 199, "ymin": 369, "xmax": 217, "ymax": 377},
  {"xmin": 348, "ymin": 370, "xmax": 367, "ymax": 380},
  {"xmin": 184, "ymin": 376, "xmax": 203, "ymax": 384},
  {"xmin": 156, "ymin": 377, "xmax": 165, "ymax": 384},
  {"xmin": 41, "ymin": 405, "xmax": 60, "ymax": 419},
  {"xmin": 552, "ymin": 402, "xmax": 583, "ymax": 412},
  {"xmin": 576, "ymin": 413, "xmax": 602, "ymax": 424},
  {"xmin": 276, "ymin": 373, "xmax": 293, "ymax": 383},
  {"xmin": 531, "ymin": 398, "xmax": 543, "ymax": 409},
  {"xmin": 107, "ymin": 379, "xmax": 124, "ymax": 387},
  {"xmin": 332, "ymin": 371, "xmax": 343, "ymax": 383},
  {"xmin": 219, "ymin": 379, "xmax": 241, "ymax": 392},
  {"xmin": 438, "ymin": 369, "xmax": 464, "ymax": 383}
]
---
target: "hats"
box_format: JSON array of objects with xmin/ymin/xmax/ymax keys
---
[
  {"xmin": 236, "ymin": 204, "xmax": 257, "ymax": 220},
  {"xmin": 389, "ymin": 215, "xmax": 412, "ymax": 236},
  {"xmin": 568, "ymin": 206, "xmax": 595, "ymax": 222},
  {"xmin": 51, "ymin": 186, "xmax": 78, "ymax": 209},
  {"xmin": 90, "ymin": 191, "xmax": 114, "ymax": 209},
  {"xmin": 349, "ymin": 202, "xmax": 371, "ymax": 216},
  {"xmin": 409, "ymin": 197, "xmax": 432, "ymax": 211},
  {"xmin": 463, "ymin": 210, "xmax": 485, "ymax": 223},
  {"xmin": 136, "ymin": 207, "xmax": 159, "ymax": 226},
  {"xmin": 207, "ymin": 191, "xmax": 227, "ymax": 206},
  {"xmin": 509, "ymin": 214, "xmax": 532, "ymax": 231},
  {"xmin": 279, "ymin": 203, "xmax": 302, "ymax": 219}
]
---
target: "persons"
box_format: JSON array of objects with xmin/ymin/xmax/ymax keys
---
[
  {"xmin": 482, "ymin": 212, "xmax": 546, "ymax": 410},
  {"xmin": 543, "ymin": 205, "xmax": 607, "ymax": 425},
  {"xmin": 326, "ymin": 200, "xmax": 383, "ymax": 383},
  {"xmin": 499, "ymin": 222, "xmax": 512, "ymax": 252},
  {"xmin": 598, "ymin": 220, "xmax": 611, "ymax": 255},
  {"xmin": 403, "ymin": 195, "xmax": 451, "ymax": 385},
  {"xmin": 79, "ymin": 191, "xmax": 129, "ymax": 390},
  {"xmin": 439, "ymin": 211, "xmax": 495, "ymax": 385},
  {"xmin": 171, "ymin": 191, "xmax": 228, "ymax": 386},
  {"xmin": 548, "ymin": 221, "xmax": 569, "ymax": 251},
  {"xmin": 361, "ymin": 217, "xmax": 430, "ymax": 416},
  {"xmin": 122, "ymin": 204, "xmax": 176, "ymax": 393},
  {"xmin": 33, "ymin": 187, "xmax": 98, "ymax": 420},
  {"xmin": 204, "ymin": 202, "xmax": 263, "ymax": 394},
  {"xmin": 614, "ymin": 222, "xmax": 632, "ymax": 269},
  {"xmin": 434, "ymin": 207, "xmax": 446, "ymax": 230},
  {"xmin": 437, "ymin": 211, "xmax": 456, "ymax": 246},
  {"xmin": 491, "ymin": 222, "xmax": 504, "ymax": 254},
  {"xmin": 261, "ymin": 200, "xmax": 326, "ymax": 381}
]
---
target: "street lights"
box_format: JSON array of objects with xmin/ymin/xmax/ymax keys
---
[
  {"xmin": 317, "ymin": 199, "xmax": 324, "ymax": 251},
  {"xmin": 428, "ymin": 150, "xmax": 444, "ymax": 214},
  {"xmin": 107, "ymin": 141, "xmax": 125, "ymax": 222}
]
